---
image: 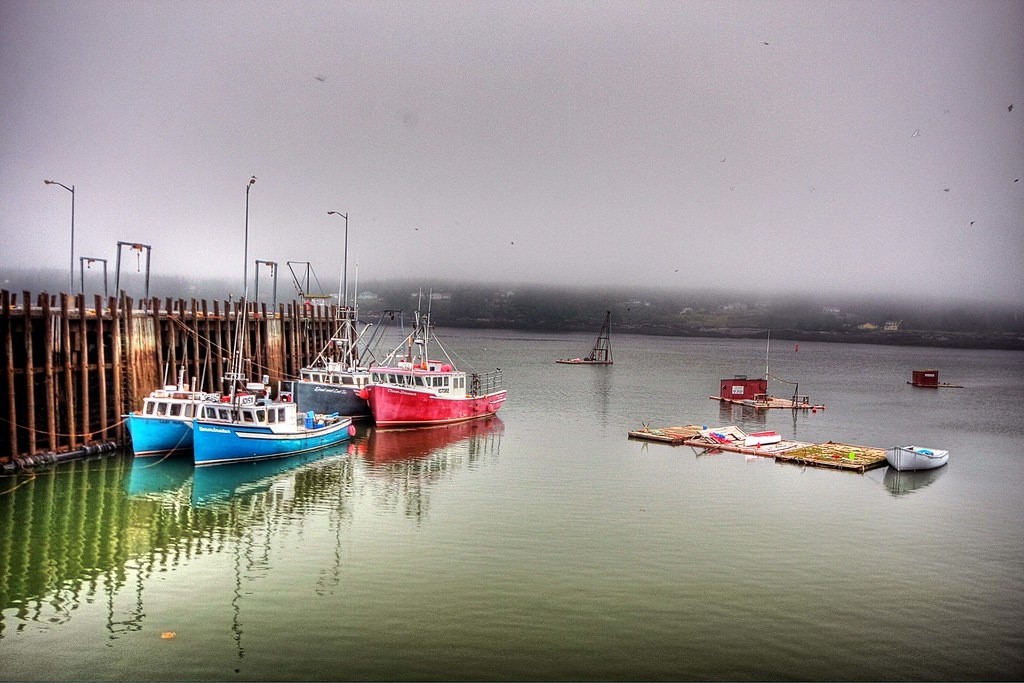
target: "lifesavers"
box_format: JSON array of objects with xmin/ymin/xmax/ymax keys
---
[
  {"xmin": 598, "ymin": 352, "xmax": 602, "ymax": 357},
  {"xmin": 348, "ymin": 424, "xmax": 356, "ymax": 436},
  {"xmin": 303, "ymin": 301, "xmax": 313, "ymax": 311},
  {"xmin": 280, "ymin": 393, "xmax": 291, "ymax": 402}
]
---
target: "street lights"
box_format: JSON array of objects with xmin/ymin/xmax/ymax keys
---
[
  {"xmin": 44, "ymin": 179, "xmax": 76, "ymax": 295},
  {"xmin": 244, "ymin": 174, "xmax": 257, "ymax": 294},
  {"xmin": 327, "ymin": 209, "xmax": 348, "ymax": 310}
]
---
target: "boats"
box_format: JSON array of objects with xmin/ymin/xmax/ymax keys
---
[
  {"xmin": 190, "ymin": 287, "xmax": 361, "ymax": 468},
  {"xmin": 885, "ymin": 444, "xmax": 950, "ymax": 472},
  {"xmin": 119, "ymin": 344, "xmax": 273, "ymax": 458},
  {"xmin": 282, "ymin": 287, "xmax": 508, "ymax": 427}
]
